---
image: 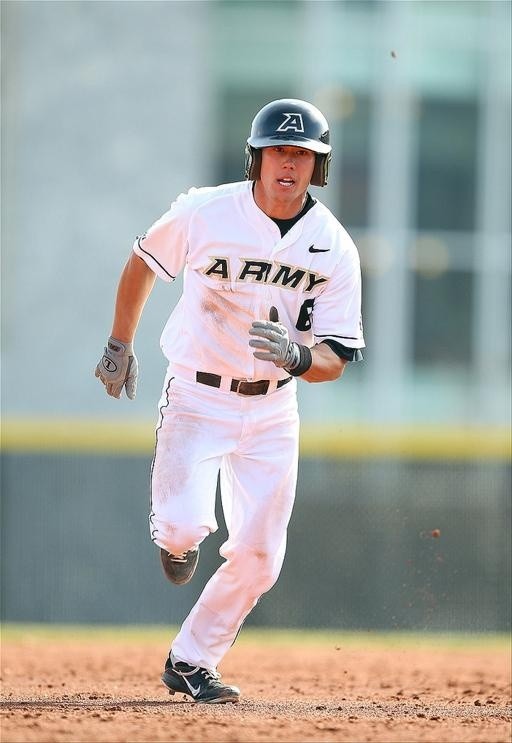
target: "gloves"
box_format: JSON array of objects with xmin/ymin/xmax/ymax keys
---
[
  {"xmin": 249, "ymin": 305, "xmax": 301, "ymax": 370},
  {"xmin": 95, "ymin": 336, "xmax": 139, "ymax": 400}
]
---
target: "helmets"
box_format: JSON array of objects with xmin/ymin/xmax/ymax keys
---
[{"xmin": 243, "ymin": 98, "xmax": 333, "ymax": 187}]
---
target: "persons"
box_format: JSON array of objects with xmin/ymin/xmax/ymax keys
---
[{"xmin": 90, "ymin": 96, "xmax": 366, "ymax": 699}]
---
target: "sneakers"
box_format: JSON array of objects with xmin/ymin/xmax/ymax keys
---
[
  {"xmin": 160, "ymin": 648, "xmax": 241, "ymax": 703},
  {"xmin": 160, "ymin": 547, "xmax": 199, "ymax": 585}
]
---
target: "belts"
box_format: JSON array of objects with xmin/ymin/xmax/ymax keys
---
[{"xmin": 195, "ymin": 370, "xmax": 292, "ymax": 397}]
